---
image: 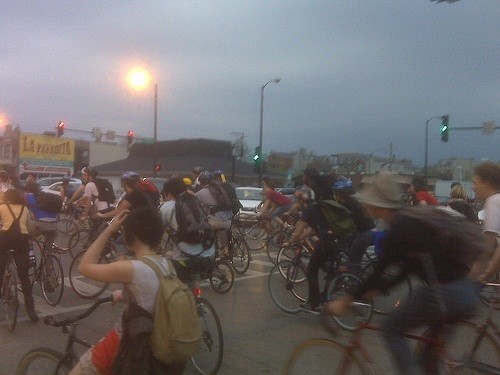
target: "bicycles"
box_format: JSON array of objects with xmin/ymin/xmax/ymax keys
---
[
  {"xmin": 0, "ymin": 170, "xmax": 500, "ymax": 375},
  {"xmin": 13, "ymin": 293, "xmax": 164, "ymax": 375}
]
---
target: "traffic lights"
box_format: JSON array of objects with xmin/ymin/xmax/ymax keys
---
[{"xmin": 440, "ymin": 114, "xmax": 449, "ymax": 141}]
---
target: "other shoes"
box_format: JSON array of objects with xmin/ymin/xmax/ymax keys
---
[
  {"xmin": 106, "ymin": 251, "xmax": 119, "ymax": 258},
  {"xmin": 299, "ymin": 302, "xmax": 321, "ymax": 314},
  {"xmin": 45, "ymin": 283, "xmax": 55, "ymax": 293},
  {"xmin": 27, "ymin": 309, "xmax": 39, "ymax": 322},
  {"xmin": 219, "ymin": 252, "xmax": 230, "ymax": 258},
  {"xmin": 29, "ymin": 262, "xmax": 36, "ymax": 268}
]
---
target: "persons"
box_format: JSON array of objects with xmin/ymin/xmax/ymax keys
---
[
  {"xmin": 0, "ymin": 161, "xmax": 243, "ymax": 322},
  {"xmin": 325, "ymin": 171, "xmax": 479, "ymax": 375},
  {"xmin": 67, "ymin": 202, "xmax": 187, "ymax": 375},
  {"xmin": 256, "ymin": 160, "xmax": 500, "ymax": 315}
]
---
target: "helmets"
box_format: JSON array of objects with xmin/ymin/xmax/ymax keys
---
[
  {"xmin": 198, "ymin": 171, "xmax": 212, "ymax": 184},
  {"xmin": 121, "ymin": 170, "xmax": 138, "ymax": 184},
  {"xmin": 214, "ymin": 168, "xmax": 222, "ymax": 177},
  {"xmin": 294, "ymin": 186, "xmax": 315, "ymax": 201},
  {"xmin": 332, "ymin": 175, "xmax": 353, "ymax": 191},
  {"xmin": 83, "ymin": 167, "xmax": 97, "ymax": 177},
  {"xmin": 183, "ymin": 178, "xmax": 193, "ymax": 186}
]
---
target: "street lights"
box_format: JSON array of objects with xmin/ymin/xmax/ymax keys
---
[
  {"xmin": 127, "ymin": 65, "xmax": 158, "ymax": 179},
  {"xmin": 256, "ymin": 77, "xmax": 281, "ymax": 189}
]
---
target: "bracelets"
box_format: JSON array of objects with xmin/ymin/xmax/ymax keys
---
[{"xmin": 288, "ymin": 241, "xmax": 291, "ymax": 246}]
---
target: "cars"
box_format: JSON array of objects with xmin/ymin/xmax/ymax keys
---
[
  {"xmin": 233, "ymin": 185, "xmax": 267, "ymax": 226},
  {"xmin": 36, "ymin": 176, "xmax": 82, "ymax": 199},
  {"xmin": 331, "ymin": 174, "xmax": 355, "ymax": 196},
  {"xmin": 276, "ymin": 187, "xmax": 296, "ymax": 202}
]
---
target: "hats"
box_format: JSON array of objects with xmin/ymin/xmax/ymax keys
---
[
  {"xmin": 163, "ymin": 177, "xmax": 186, "ymax": 195},
  {"xmin": 360, "ymin": 171, "xmax": 404, "ymax": 211}
]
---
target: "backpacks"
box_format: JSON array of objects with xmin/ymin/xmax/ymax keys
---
[
  {"xmin": 409, "ymin": 206, "xmax": 484, "ymax": 258},
  {"xmin": 89, "ymin": 178, "xmax": 116, "ymax": 207},
  {"xmin": 167, "ymin": 191, "xmax": 212, "ymax": 244},
  {"xmin": 136, "ymin": 257, "xmax": 203, "ymax": 364},
  {"xmin": 35, "ymin": 191, "xmax": 63, "ymax": 212},
  {"xmin": 203, "ymin": 181, "xmax": 238, "ymax": 215},
  {"xmin": 313, "ymin": 200, "xmax": 357, "ymax": 238}
]
